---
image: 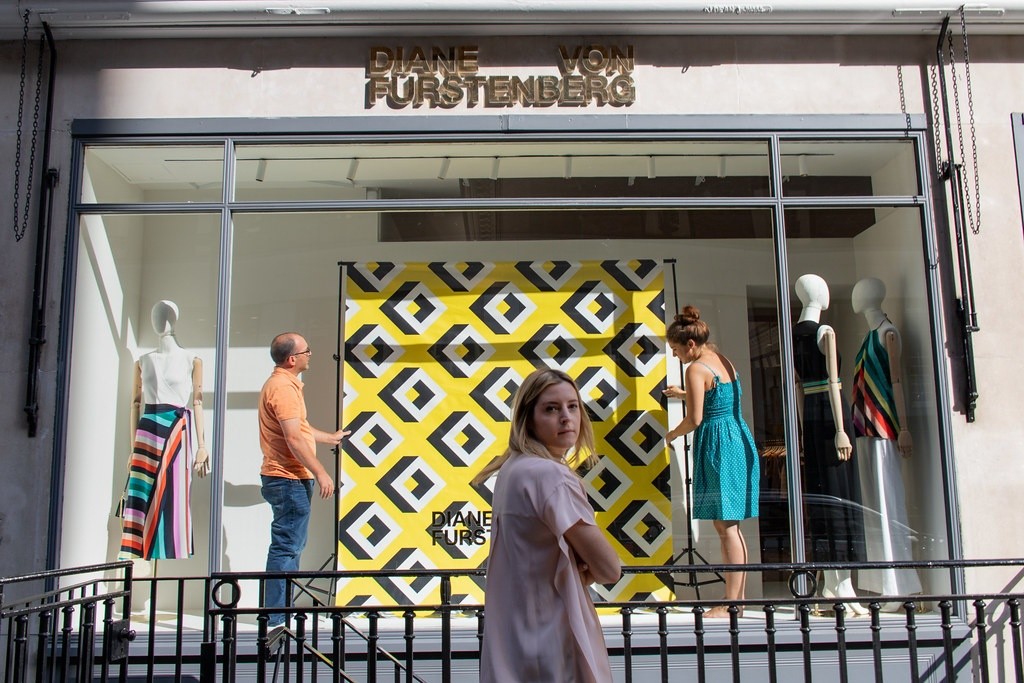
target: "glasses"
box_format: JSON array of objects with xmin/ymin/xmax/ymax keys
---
[{"xmin": 287, "ymin": 347, "xmax": 310, "ymax": 362}]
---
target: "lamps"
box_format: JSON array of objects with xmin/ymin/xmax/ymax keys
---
[{"xmin": 256, "ymin": 157, "xmax": 809, "ymax": 179}]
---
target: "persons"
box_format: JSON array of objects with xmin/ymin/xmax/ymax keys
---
[
  {"xmin": 662, "ymin": 305, "xmax": 760, "ymax": 618},
  {"xmin": 849, "ymin": 277, "xmax": 926, "ymax": 614},
  {"xmin": 109, "ymin": 300, "xmax": 210, "ymax": 623},
  {"xmin": 480, "ymin": 367, "xmax": 621, "ymax": 683},
  {"xmin": 794, "ymin": 273, "xmax": 872, "ymax": 617},
  {"xmin": 259, "ymin": 332, "xmax": 351, "ymax": 633}
]
